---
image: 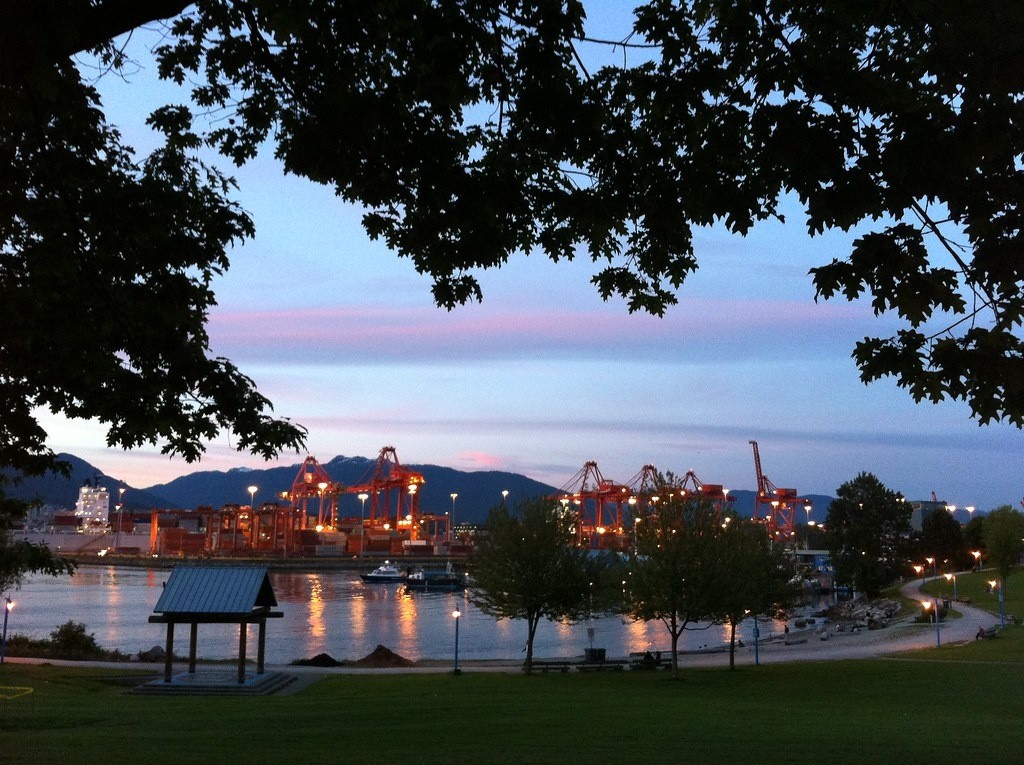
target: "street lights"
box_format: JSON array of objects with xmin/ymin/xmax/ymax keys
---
[
  {"xmin": 116, "ymin": 488, "xmax": 126, "ymax": 551},
  {"xmin": 989, "ymin": 576, "xmax": 1005, "ymax": 629},
  {"xmin": 914, "ymin": 562, "xmax": 925, "ymax": 586},
  {"xmin": 0, "ymin": 593, "xmax": 14, "ymax": 662},
  {"xmin": 971, "ymin": 548, "xmax": 982, "ymax": 574},
  {"xmin": 113, "ymin": 505, "xmax": 120, "ymax": 555},
  {"xmin": 246, "ymin": 486, "xmax": 259, "ymax": 507},
  {"xmin": 927, "ymin": 555, "xmax": 936, "ymax": 580},
  {"xmin": 944, "ymin": 573, "xmax": 958, "ymax": 603},
  {"xmin": 450, "ymin": 603, "xmax": 461, "ymax": 675},
  {"xmin": 451, "ymin": 493, "xmax": 458, "ymax": 542},
  {"xmin": 498, "ymin": 483, "xmax": 978, "ymax": 548},
  {"xmin": 923, "ymin": 594, "xmax": 941, "ymax": 647},
  {"xmin": 359, "ymin": 494, "xmax": 368, "ymax": 558}
]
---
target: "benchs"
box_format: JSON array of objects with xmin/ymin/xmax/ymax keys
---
[
  {"xmin": 951, "ymin": 596, "xmax": 960, "ymax": 601},
  {"xmin": 959, "ymin": 596, "xmax": 971, "ymax": 603},
  {"xmin": 521, "ymin": 661, "xmax": 575, "ymax": 673},
  {"xmin": 575, "ymin": 660, "xmax": 628, "ymax": 671},
  {"xmin": 629, "ymin": 658, "xmax": 673, "ymax": 670},
  {"xmin": 981, "ymin": 627, "xmax": 996, "ymax": 640}
]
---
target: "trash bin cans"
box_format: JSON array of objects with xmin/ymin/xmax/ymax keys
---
[{"xmin": 584, "ymin": 647, "xmax": 606, "ymax": 661}]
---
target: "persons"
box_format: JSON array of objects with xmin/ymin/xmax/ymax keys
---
[
  {"xmin": 946, "ymin": 592, "xmax": 952, "ymax": 608},
  {"xmin": 642, "ymin": 651, "xmax": 661, "ymax": 670},
  {"xmin": 975, "ymin": 625, "xmax": 985, "ymax": 639},
  {"xmin": 738, "ymin": 601, "xmax": 871, "ymax": 646}
]
